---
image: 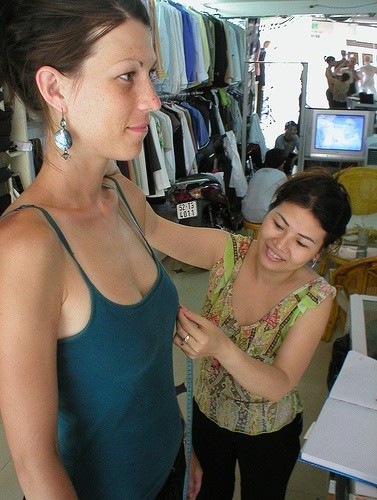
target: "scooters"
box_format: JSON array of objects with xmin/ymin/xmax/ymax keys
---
[{"xmin": 164, "ymin": 133, "xmax": 236, "ymax": 229}]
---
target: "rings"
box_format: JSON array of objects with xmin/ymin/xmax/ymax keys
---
[{"xmin": 180, "ymin": 334, "xmax": 191, "ymax": 346}]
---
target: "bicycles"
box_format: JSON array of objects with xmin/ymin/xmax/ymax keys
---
[{"xmin": 260, "ymin": 86, "xmax": 276, "ymax": 132}]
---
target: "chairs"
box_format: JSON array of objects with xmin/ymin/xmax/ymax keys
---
[{"xmin": 310, "ymin": 166, "xmax": 377, "ymax": 342}]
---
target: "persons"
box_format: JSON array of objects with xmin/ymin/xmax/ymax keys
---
[
  {"xmin": 0, "ymin": 0, "xmax": 203, "ymax": 500},
  {"xmin": 336, "ymin": 118, "xmax": 360, "ymax": 150},
  {"xmin": 274, "ymin": 121, "xmax": 300, "ymax": 176},
  {"xmin": 241, "ymin": 147, "xmax": 289, "ymax": 225},
  {"xmin": 324, "ymin": 50, "xmax": 377, "ymax": 109},
  {"xmin": 100, "ymin": 172, "xmax": 352, "ymax": 500}
]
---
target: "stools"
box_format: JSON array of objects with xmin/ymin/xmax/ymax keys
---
[{"xmin": 243, "ymin": 219, "xmax": 262, "ymax": 241}]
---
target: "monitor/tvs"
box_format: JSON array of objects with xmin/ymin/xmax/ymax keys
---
[{"xmin": 305, "ymin": 108, "xmax": 370, "ymax": 162}]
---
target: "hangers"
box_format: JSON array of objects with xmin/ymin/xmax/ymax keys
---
[{"xmin": 155, "ymin": 88, "xmax": 203, "ymax": 106}]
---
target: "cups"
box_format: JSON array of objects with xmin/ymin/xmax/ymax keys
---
[{"xmin": 357, "ymin": 230, "xmax": 369, "ymax": 246}]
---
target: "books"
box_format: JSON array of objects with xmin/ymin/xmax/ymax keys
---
[{"xmin": 297, "ymin": 350, "xmax": 377, "ymax": 500}]
